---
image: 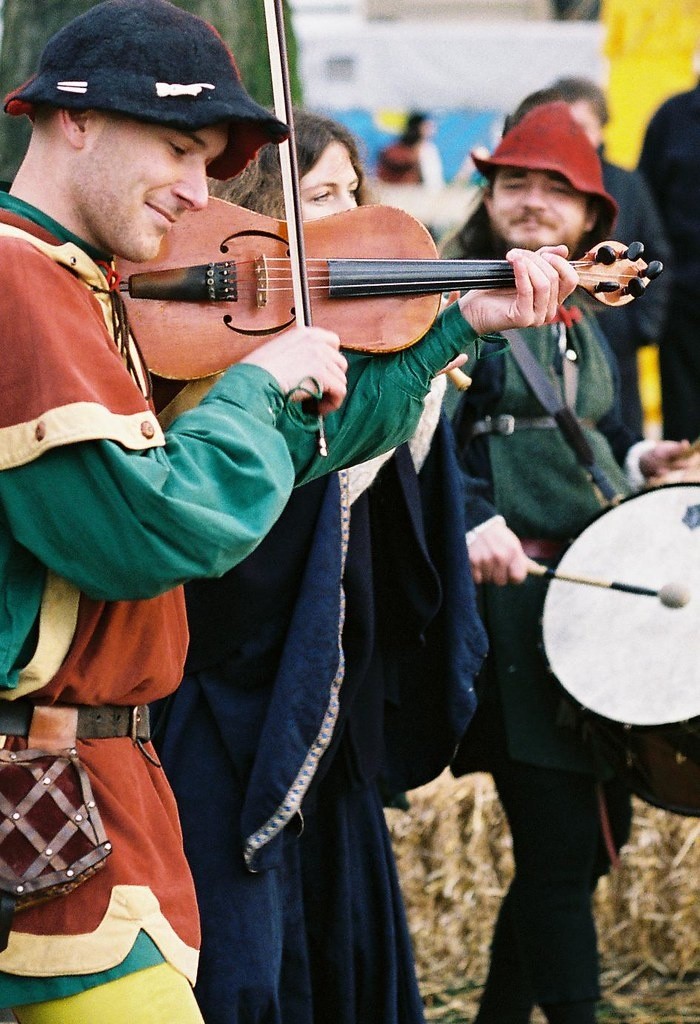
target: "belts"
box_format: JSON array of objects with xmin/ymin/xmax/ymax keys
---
[{"xmin": 0, "ymin": 695, "xmax": 152, "ymax": 745}]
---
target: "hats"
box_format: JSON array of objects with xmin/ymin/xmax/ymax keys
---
[
  {"xmin": 2, "ymin": 0, "xmax": 289, "ymax": 181},
  {"xmin": 470, "ymin": 105, "xmax": 620, "ymax": 261}
]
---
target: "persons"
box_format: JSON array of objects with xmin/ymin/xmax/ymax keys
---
[
  {"xmin": 149, "ymin": 110, "xmax": 488, "ymax": 1024},
  {"xmin": 0, "ymin": 0, "xmax": 580, "ymax": 1024},
  {"xmin": 438, "ymin": 98, "xmax": 700, "ymax": 1018},
  {"xmin": 634, "ymin": 73, "xmax": 700, "ymax": 445},
  {"xmin": 498, "ymin": 75, "xmax": 678, "ymax": 445},
  {"xmin": 398, "ymin": 111, "xmax": 445, "ymax": 188}
]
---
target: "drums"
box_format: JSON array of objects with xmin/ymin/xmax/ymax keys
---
[{"xmin": 531, "ymin": 475, "xmax": 700, "ymax": 823}]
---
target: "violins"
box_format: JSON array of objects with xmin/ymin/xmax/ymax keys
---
[{"xmin": 107, "ymin": 187, "xmax": 666, "ymax": 385}]
